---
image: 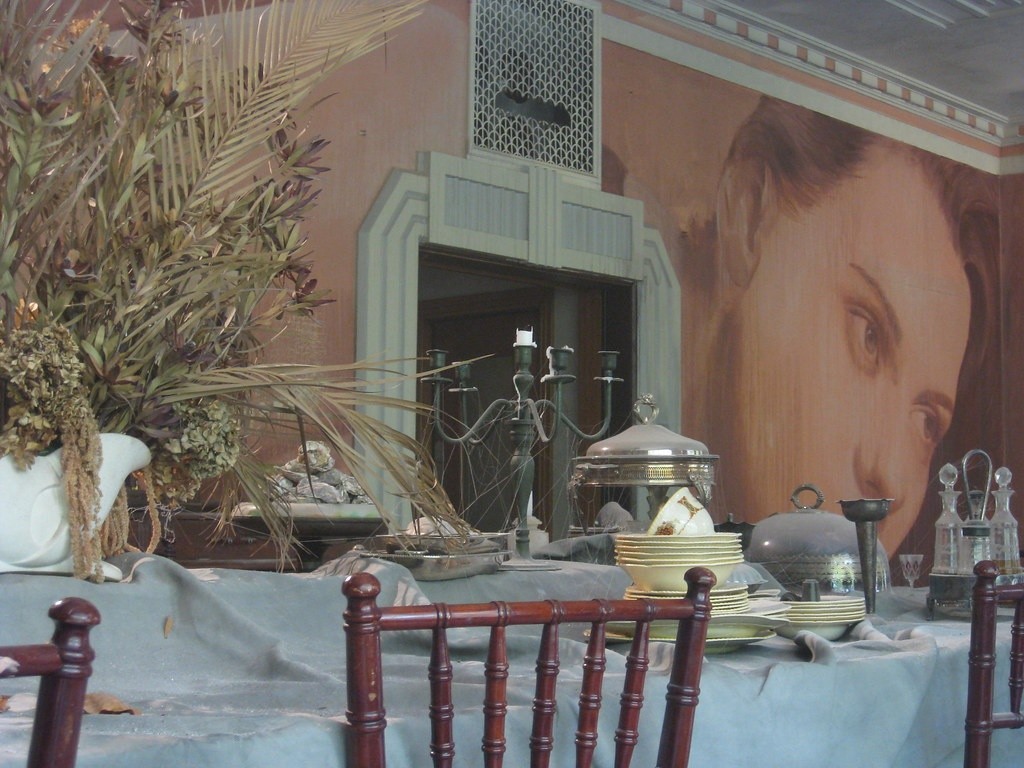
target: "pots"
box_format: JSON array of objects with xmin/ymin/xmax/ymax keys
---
[
  {"xmin": 744, "ymin": 483, "xmax": 892, "ymax": 595},
  {"xmin": 568, "ymin": 395, "xmax": 720, "ymax": 538}
]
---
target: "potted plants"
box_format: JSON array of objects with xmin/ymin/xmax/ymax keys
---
[{"xmin": 2, "ymin": 0, "xmax": 502, "ymax": 585}]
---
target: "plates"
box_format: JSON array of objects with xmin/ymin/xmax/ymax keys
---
[
  {"xmin": 585, "ymin": 583, "xmax": 867, "ymax": 657},
  {"xmin": 356, "ymin": 516, "xmax": 513, "ymax": 581}
]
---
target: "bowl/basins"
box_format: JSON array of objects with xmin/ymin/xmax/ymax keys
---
[
  {"xmin": 645, "ymin": 485, "xmax": 715, "ymax": 534},
  {"xmin": 728, "ymin": 563, "xmax": 768, "ymax": 593},
  {"xmin": 613, "ymin": 531, "xmax": 743, "ymax": 590}
]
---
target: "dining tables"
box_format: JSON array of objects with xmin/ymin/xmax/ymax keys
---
[{"xmin": 0, "ymin": 557, "xmax": 1024, "ymax": 768}]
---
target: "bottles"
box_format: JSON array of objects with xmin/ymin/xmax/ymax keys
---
[
  {"xmin": 932, "ymin": 462, "xmax": 964, "ymax": 572},
  {"xmin": 987, "ymin": 467, "xmax": 1023, "ymax": 603},
  {"xmin": 966, "ymin": 490, "xmax": 988, "ymax": 522},
  {"xmin": 959, "ymin": 524, "xmax": 993, "ymax": 575}
]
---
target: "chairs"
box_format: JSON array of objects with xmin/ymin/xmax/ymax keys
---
[
  {"xmin": 961, "ymin": 559, "xmax": 1024, "ymax": 768},
  {"xmin": 342, "ymin": 565, "xmax": 717, "ymax": 768},
  {"xmin": 1, "ymin": 597, "xmax": 101, "ymax": 767}
]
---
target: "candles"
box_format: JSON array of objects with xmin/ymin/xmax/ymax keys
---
[{"xmin": 513, "ymin": 325, "xmax": 537, "ymax": 348}]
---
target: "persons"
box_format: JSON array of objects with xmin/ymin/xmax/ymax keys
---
[{"xmin": 602, "ymin": 92, "xmax": 1004, "ymax": 591}]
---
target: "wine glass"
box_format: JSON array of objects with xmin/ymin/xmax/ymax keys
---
[{"xmin": 897, "ymin": 554, "xmax": 923, "ymax": 597}]
